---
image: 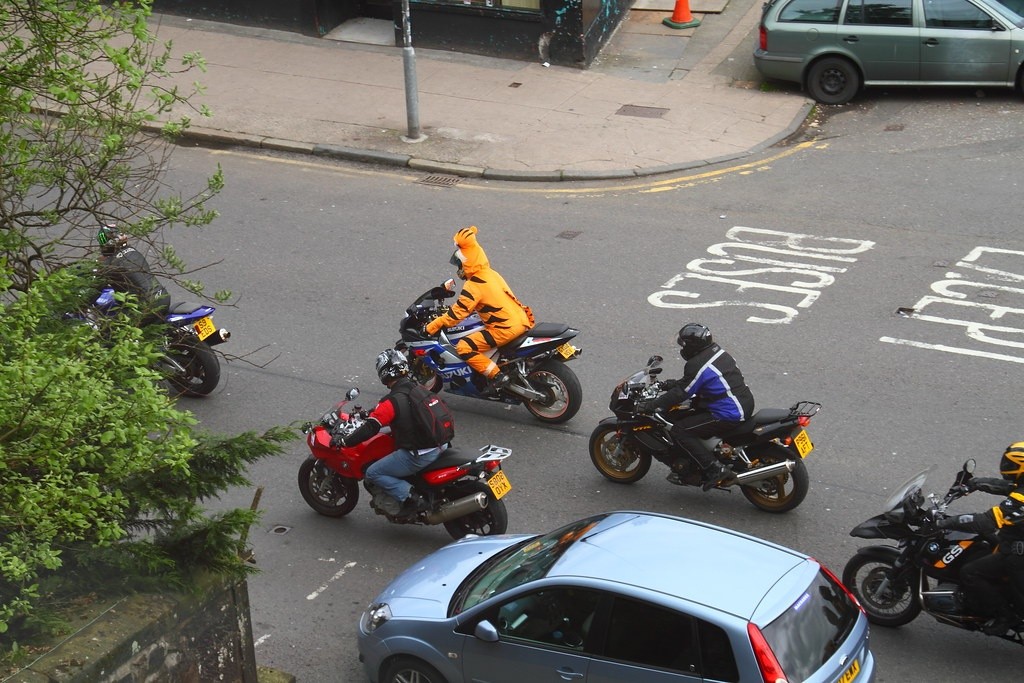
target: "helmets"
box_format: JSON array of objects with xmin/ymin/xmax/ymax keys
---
[
  {"xmin": 96, "ymin": 223, "xmax": 128, "ymax": 256},
  {"xmin": 999, "ymin": 440, "xmax": 1024, "ymax": 484},
  {"xmin": 449, "ymin": 250, "xmax": 467, "ymax": 280},
  {"xmin": 677, "ymin": 323, "xmax": 714, "ymax": 360},
  {"xmin": 376, "ymin": 349, "xmax": 410, "ymax": 385}
]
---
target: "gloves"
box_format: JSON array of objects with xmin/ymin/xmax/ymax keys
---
[
  {"xmin": 654, "ymin": 380, "xmax": 668, "ymax": 391},
  {"xmin": 933, "ymin": 513, "xmax": 950, "ymax": 529},
  {"xmin": 961, "ymin": 476, "xmax": 979, "ymax": 493},
  {"xmin": 636, "ymin": 401, "xmax": 655, "ymax": 415},
  {"xmin": 329, "ymin": 435, "xmax": 345, "ymax": 447},
  {"xmin": 420, "ymin": 325, "xmax": 429, "ymax": 337}
]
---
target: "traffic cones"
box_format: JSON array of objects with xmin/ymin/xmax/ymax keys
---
[{"xmin": 661, "ymin": 0, "xmax": 701, "ymax": 28}]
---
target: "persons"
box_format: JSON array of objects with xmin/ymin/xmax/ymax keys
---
[
  {"xmin": 920, "ymin": 442, "xmax": 1024, "ymax": 636},
  {"xmin": 72, "ymin": 223, "xmax": 171, "ymax": 351},
  {"xmin": 422, "ymin": 228, "xmax": 535, "ymax": 396},
  {"xmin": 634, "ymin": 322, "xmax": 754, "ymax": 491},
  {"xmin": 329, "ymin": 349, "xmax": 447, "ymax": 520}
]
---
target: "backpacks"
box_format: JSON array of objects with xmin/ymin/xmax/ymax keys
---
[{"xmin": 389, "ymin": 384, "xmax": 455, "ymax": 447}]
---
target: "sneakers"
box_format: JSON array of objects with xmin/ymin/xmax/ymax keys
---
[
  {"xmin": 393, "ymin": 494, "xmax": 428, "ymax": 523},
  {"xmin": 481, "ymin": 370, "xmax": 509, "ymax": 394},
  {"xmin": 983, "ymin": 601, "xmax": 1022, "ymax": 634},
  {"xmin": 702, "ymin": 459, "xmax": 731, "ymax": 491}
]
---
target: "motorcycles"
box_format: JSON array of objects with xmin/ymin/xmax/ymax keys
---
[
  {"xmin": 298, "ymin": 387, "xmax": 513, "ymax": 539},
  {"xmin": 66, "ymin": 289, "xmax": 232, "ymax": 399},
  {"xmin": 843, "ymin": 458, "xmax": 1024, "ymax": 645},
  {"xmin": 395, "ymin": 278, "xmax": 583, "ymax": 425},
  {"xmin": 589, "ymin": 355, "xmax": 822, "ymax": 512}
]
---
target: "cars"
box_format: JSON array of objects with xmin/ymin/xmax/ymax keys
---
[
  {"xmin": 752, "ymin": 0, "xmax": 1024, "ymax": 106},
  {"xmin": 357, "ymin": 509, "xmax": 874, "ymax": 683}
]
